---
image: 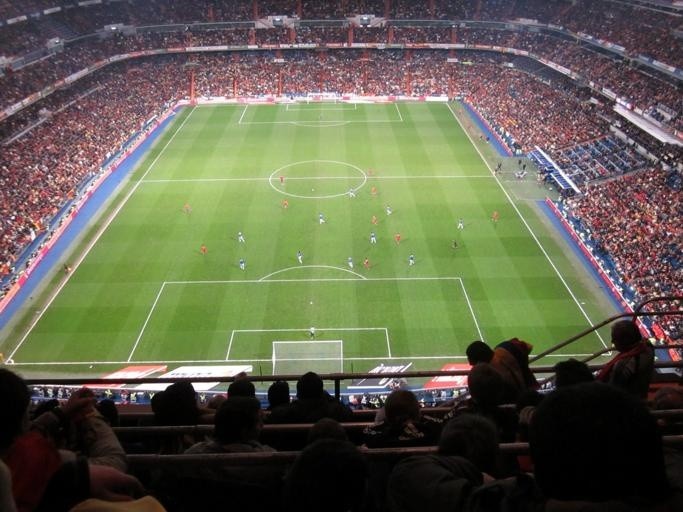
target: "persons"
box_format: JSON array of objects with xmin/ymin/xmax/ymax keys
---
[{"xmin": 0, "ymin": 0, "xmax": 682, "ymax": 512}]
